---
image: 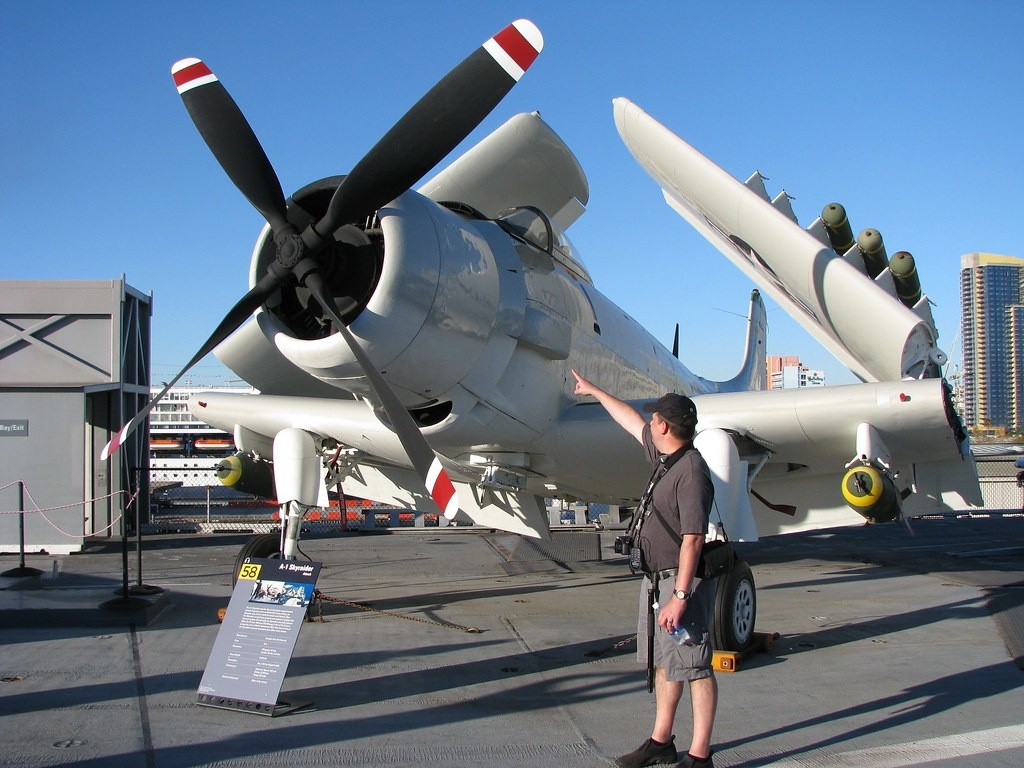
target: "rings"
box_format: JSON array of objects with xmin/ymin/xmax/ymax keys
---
[{"xmin": 667, "ymin": 621, "xmax": 671, "ymax": 623}]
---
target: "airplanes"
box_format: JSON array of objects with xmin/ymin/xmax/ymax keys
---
[{"xmin": 98, "ymin": 17, "xmax": 984, "ymax": 623}]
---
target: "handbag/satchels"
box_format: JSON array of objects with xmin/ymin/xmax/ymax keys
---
[{"xmin": 694, "ymin": 540, "xmax": 736, "ymax": 580}]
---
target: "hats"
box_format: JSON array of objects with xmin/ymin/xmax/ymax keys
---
[{"xmin": 642, "ymin": 393, "xmax": 697, "ymax": 428}]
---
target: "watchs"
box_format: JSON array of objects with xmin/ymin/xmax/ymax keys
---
[{"xmin": 673, "ymin": 588, "xmax": 689, "ymax": 600}]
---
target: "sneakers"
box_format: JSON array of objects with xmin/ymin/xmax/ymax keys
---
[
  {"xmin": 614, "ymin": 734, "xmax": 677, "ymax": 768},
  {"xmin": 675, "ymin": 750, "xmax": 714, "ymax": 768}
]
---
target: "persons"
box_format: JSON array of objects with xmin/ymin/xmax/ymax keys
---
[
  {"xmin": 251, "ymin": 579, "xmax": 278, "ymax": 603},
  {"xmin": 570, "ymin": 369, "xmax": 715, "ymax": 768}
]
